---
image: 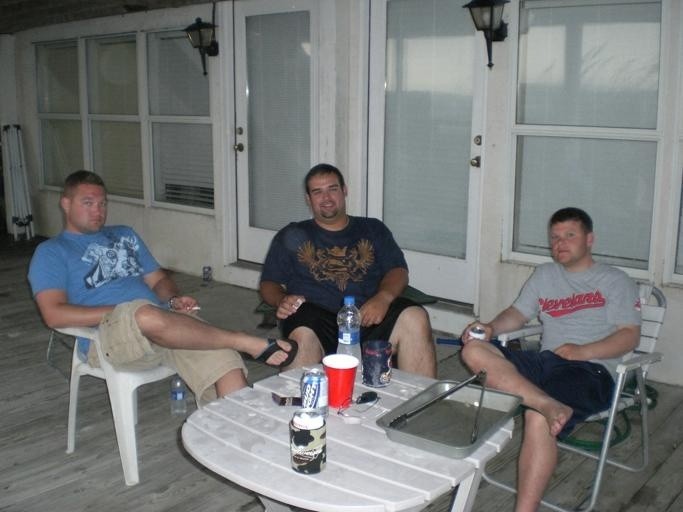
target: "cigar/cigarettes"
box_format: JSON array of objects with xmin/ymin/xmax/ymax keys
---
[{"xmin": 187, "ymin": 306, "xmax": 202, "ymax": 310}]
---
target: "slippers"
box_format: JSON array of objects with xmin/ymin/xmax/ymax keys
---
[{"xmin": 241, "ymin": 337, "xmax": 297, "ymax": 367}]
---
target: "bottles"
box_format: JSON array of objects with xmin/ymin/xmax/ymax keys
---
[
  {"xmin": 335, "ymin": 295, "xmax": 363, "ymax": 386},
  {"xmin": 169, "ymin": 375, "xmax": 187, "ymax": 416}
]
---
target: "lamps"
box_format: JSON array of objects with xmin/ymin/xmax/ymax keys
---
[
  {"xmin": 181, "ymin": 17, "xmax": 218, "ymax": 75},
  {"xmin": 462, "ymin": 0, "xmax": 511, "ymax": 72}
]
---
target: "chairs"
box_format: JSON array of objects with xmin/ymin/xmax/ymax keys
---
[
  {"xmin": 482, "ymin": 283, "xmax": 666, "ymax": 512},
  {"xmin": 54, "ymin": 327, "xmax": 178, "ymax": 486}
]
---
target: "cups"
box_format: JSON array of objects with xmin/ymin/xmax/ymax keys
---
[{"xmin": 321, "ymin": 354, "xmax": 361, "ymax": 409}]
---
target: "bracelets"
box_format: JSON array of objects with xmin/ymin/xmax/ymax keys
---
[{"xmin": 167, "ymin": 295, "xmax": 177, "ymax": 309}]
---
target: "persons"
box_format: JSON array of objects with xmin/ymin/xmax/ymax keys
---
[
  {"xmin": 26, "ymin": 168, "xmax": 298, "ymax": 410},
  {"xmin": 459, "ymin": 206, "xmax": 643, "ymax": 512},
  {"xmin": 257, "ymin": 162, "xmax": 439, "ymax": 380}
]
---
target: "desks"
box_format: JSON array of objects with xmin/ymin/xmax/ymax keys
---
[{"xmin": 181, "ymin": 364, "xmax": 515, "ymax": 512}]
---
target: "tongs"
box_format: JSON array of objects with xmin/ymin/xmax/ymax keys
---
[{"xmin": 387, "ymin": 368, "xmax": 488, "ymax": 446}]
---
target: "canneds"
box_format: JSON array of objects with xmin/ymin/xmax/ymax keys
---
[
  {"xmin": 288, "ymin": 410, "xmax": 327, "ymax": 475},
  {"xmin": 300, "ymin": 369, "xmax": 330, "ymax": 419}
]
